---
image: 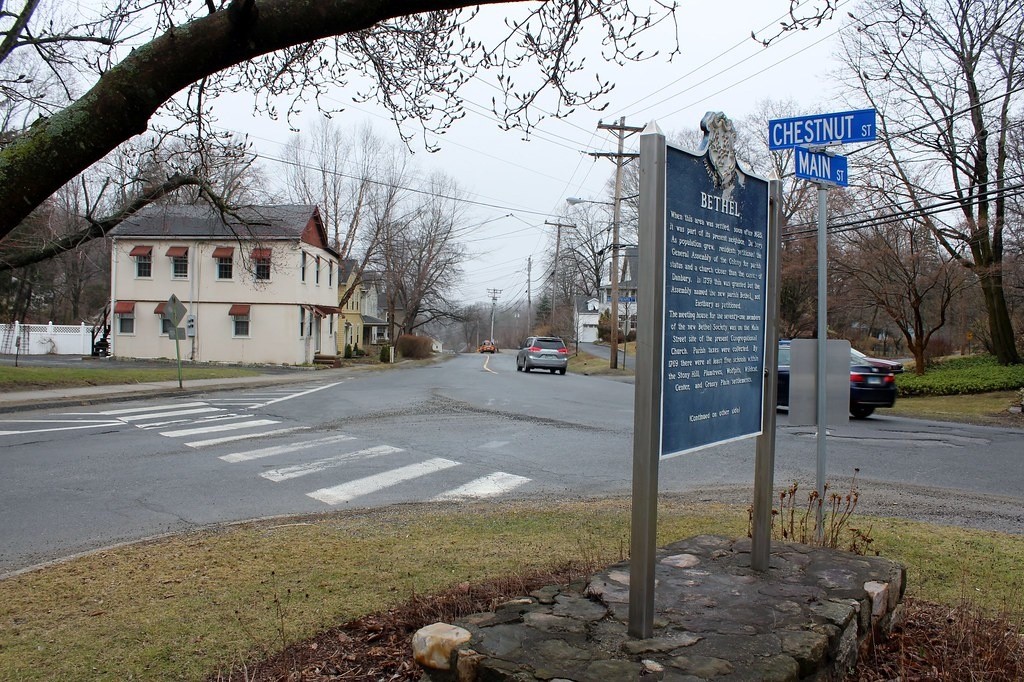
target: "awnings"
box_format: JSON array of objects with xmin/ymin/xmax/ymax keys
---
[
  {"xmin": 212, "ymin": 247, "xmax": 234, "ymax": 262},
  {"xmin": 129, "ymin": 246, "xmax": 152, "ymax": 259},
  {"xmin": 301, "ymin": 305, "xmax": 342, "ymax": 323},
  {"xmin": 165, "ymin": 247, "xmax": 188, "ymax": 263},
  {"xmin": 228, "ymin": 305, "xmax": 250, "ymax": 316},
  {"xmin": 301, "ymin": 250, "xmax": 344, "ymax": 274},
  {"xmin": 154, "ymin": 303, "xmax": 167, "ymax": 314},
  {"xmin": 250, "ymin": 249, "xmax": 272, "ymax": 260},
  {"xmin": 114, "ymin": 302, "xmax": 134, "ymax": 313}
]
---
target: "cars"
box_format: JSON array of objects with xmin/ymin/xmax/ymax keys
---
[
  {"xmin": 849, "ymin": 347, "xmax": 905, "ymax": 375},
  {"xmin": 777, "ymin": 345, "xmax": 897, "ymax": 416}
]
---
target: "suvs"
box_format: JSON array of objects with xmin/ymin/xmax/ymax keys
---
[
  {"xmin": 516, "ymin": 334, "xmax": 568, "ymax": 375},
  {"xmin": 480, "ymin": 341, "xmax": 495, "ymax": 354}
]
